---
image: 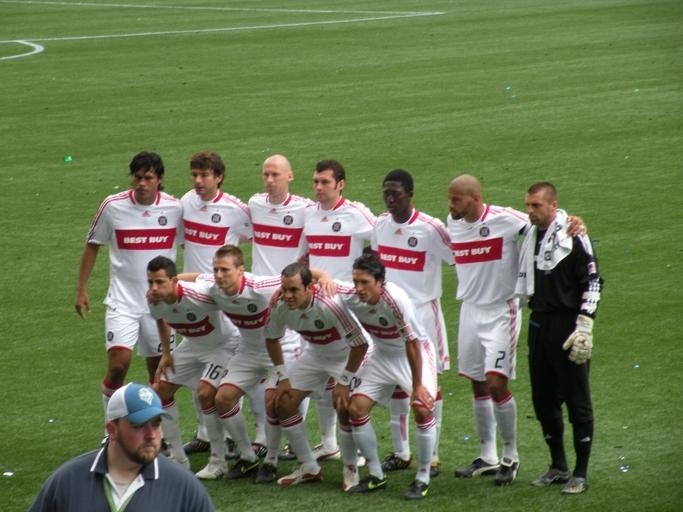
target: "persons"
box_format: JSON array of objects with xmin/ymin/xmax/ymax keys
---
[
  {"xmin": 27, "ymin": 381, "xmax": 218, "ymax": 512},
  {"xmin": 72, "ymin": 150, "xmax": 458, "ymax": 498},
  {"xmin": 447, "ymin": 173, "xmax": 587, "ymax": 488},
  {"xmin": 512, "ymin": 182, "xmax": 604, "ymax": 496}
]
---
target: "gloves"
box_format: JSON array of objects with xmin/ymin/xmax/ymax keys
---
[{"xmin": 563, "ymin": 314, "xmax": 594, "ymax": 365}]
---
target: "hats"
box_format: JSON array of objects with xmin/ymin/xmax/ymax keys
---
[{"xmin": 107, "ymin": 382, "xmax": 172, "ymax": 426}]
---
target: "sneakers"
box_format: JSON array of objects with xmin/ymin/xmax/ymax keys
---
[
  {"xmin": 455, "ymin": 457, "xmax": 500, "ymax": 478},
  {"xmin": 167, "ymin": 437, "xmax": 441, "ymax": 500},
  {"xmin": 495, "ymin": 457, "xmax": 520, "ymax": 485},
  {"xmin": 530, "ymin": 467, "xmax": 570, "ymax": 487},
  {"xmin": 561, "ymin": 478, "xmax": 588, "ymax": 494}
]
